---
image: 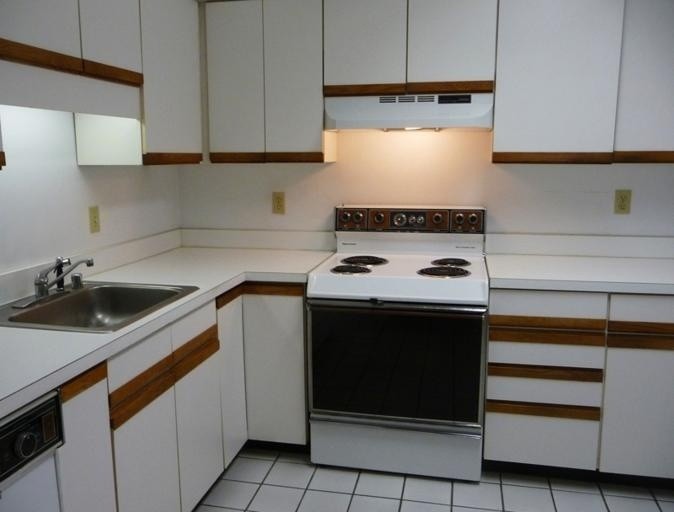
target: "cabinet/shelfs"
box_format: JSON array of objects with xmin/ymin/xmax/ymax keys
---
[
  {"xmin": 1, "ymin": 3, "xmax": 144, "ymax": 87},
  {"xmin": 217, "ymin": 298, "xmax": 249, "ymax": 468},
  {"xmin": 481, "ymin": 288, "xmax": 609, "ymax": 472},
  {"xmin": 107, "ymin": 299, "xmax": 226, "ymax": 512},
  {"xmin": 242, "ymin": 284, "xmax": 310, "ymax": 447},
  {"xmin": 199, "ymin": 2, "xmax": 324, "ymax": 161},
  {"xmin": 495, "ymin": 0, "xmax": 674, "ymax": 163},
  {"xmin": 599, "ymin": 294, "xmax": 673, "ymax": 480},
  {"xmin": 323, "ymin": 3, "xmax": 495, "ymax": 96},
  {"xmin": 0, "ymin": 364, "xmax": 118, "ymax": 512},
  {"xmin": 145, "ymin": 1, "xmax": 209, "ymax": 164}
]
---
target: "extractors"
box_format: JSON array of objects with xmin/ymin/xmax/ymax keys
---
[{"xmin": 324, "ymin": 94, "xmax": 493, "ymax": 131}]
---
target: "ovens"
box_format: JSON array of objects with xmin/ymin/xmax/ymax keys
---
[{"xmin": 305, "ymin": 298, "xmax": 489, "ymax": 484}]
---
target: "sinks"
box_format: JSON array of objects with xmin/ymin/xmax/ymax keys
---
[{"xmin": 0, "ymin": 280, "xmax": 195, "ymax": 334}]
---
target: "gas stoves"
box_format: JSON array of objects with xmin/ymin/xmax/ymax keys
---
[{"xmin": 306, "ymin": 207, "xmax": 490, "ymax": 306}]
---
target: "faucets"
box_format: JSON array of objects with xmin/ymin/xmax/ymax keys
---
[
  {"xmin": 71, "ymin": 273, "xmax": 86, "ymax": 290},
  {"xmin": 11, "ymin": 257, "xmax": 94, "ymax": 309}
]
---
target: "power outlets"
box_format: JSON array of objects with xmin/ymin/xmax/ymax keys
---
[
  {"xmin": 614, "ymin": 190, "xmax": 632, "ymax": 214},
  {"xmin": 271, "ymin": 192, "xmax": 285, "ymax": 215},
  {"xmin": 88, "ymin": 206, "xmax": 100, "ymax": 233}
]
